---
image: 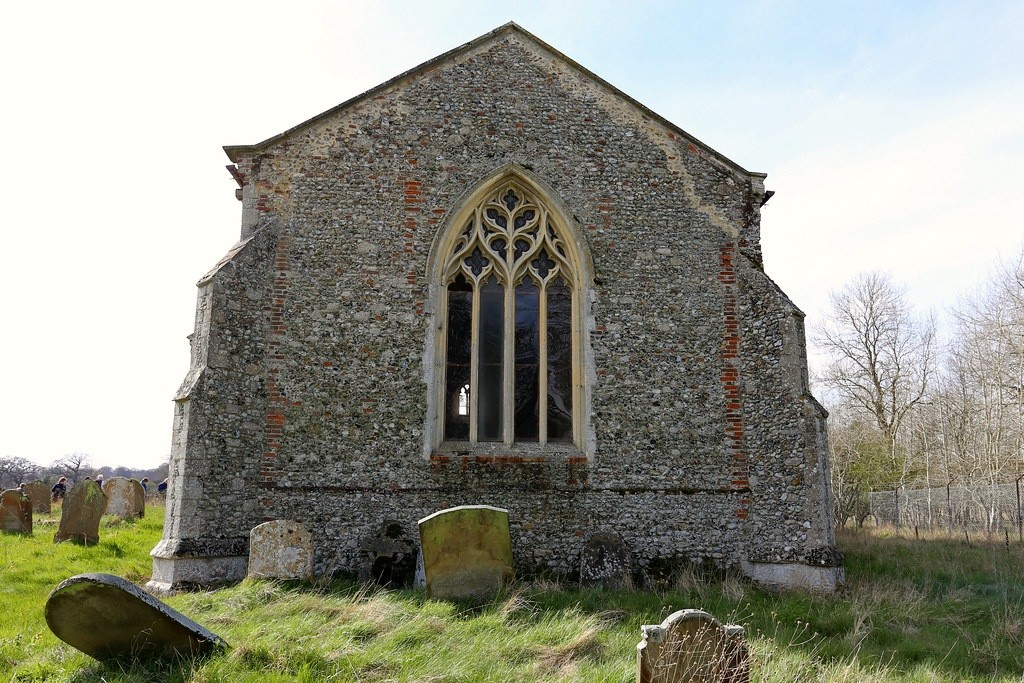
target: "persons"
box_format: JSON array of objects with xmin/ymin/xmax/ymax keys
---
[
  {"xmin": 50, "ymin": 477, "xmax": 67, "ymax": 503},
  {"xmin": 16, "ymin": 483, "xmax": 27, "ymax": 494},
  {"xmin": 158, "ymin": 478, "xmax": 168, "ymax": 495},
  {"xmin": 140, "ymin": 477, "xmax": 148, "ymax": 492},
  {"xmin": 84, "ymin": 474, "xmax": 103, "ymax": 487}
]
